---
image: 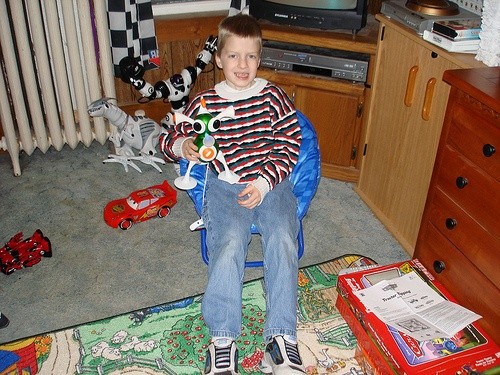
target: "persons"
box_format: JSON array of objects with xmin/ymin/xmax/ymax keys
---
[{"xmin": 159, "ymin": 14, "xmax": 305, "ymax": 375}]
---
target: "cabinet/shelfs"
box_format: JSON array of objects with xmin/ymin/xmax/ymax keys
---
[
  {"xmin": 356, "ymin": 14, "xmax": 487, "ymax": 259},
  {"xmin": 255, "ymin": 18, "xmax": 379, "ymax": 183},
  {"xmin": 413, "ymin": 67, "xmax": 500, "ymax": 346}
]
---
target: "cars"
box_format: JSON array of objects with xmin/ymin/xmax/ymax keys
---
[
  {"xmin": 103, "ymin": 180, "xmax": 178, "ymax": 230},
  {"xmin": 0, "ymin": 228, "xmax": 52, "ymax": 276}
]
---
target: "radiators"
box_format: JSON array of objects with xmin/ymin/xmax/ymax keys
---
[{"xmin": 0, "ymin": 0, "xmax": 121, "ymax": 177}]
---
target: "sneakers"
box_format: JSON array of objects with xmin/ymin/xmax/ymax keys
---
[
  {"xmin": 204, "ymin": 340, "xmax": 239, "ymax": 375},
  {"xmin": 258, "ymin": 334, "xmax": 306, "ymax": 375}
]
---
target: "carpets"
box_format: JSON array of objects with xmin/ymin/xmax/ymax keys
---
[{"xmin": 0, "ymin": 254, "xmax": 378, "ymax": 375}]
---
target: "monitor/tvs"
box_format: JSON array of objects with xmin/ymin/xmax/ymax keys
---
[{"xmin": 250, "ymin": 0, "xmax": 369, "ymax": 34}]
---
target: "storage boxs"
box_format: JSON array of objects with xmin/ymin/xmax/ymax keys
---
[{"xmin": 334, "ymin": 259, "xmax": 500, "ymax": 375}]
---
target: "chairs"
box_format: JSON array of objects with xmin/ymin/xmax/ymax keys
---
[{"xmin": 180, "ymin": 110, "xmax": 321, "ymax": 268}]
